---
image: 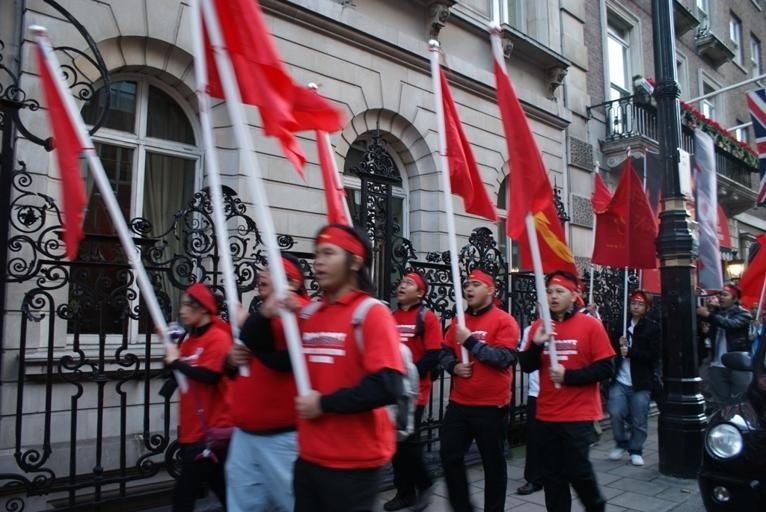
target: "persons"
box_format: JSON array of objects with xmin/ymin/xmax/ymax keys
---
[
  {"xmin": 694, "ymin": 281, "xmax": 766, "ymax": 371},
  {"xmin": 438, "ymin": 267, "xmax": 521, "ymax": 509},
  {"xmin": 383, "ymin": 271, "xmax": 442, "ymax": 511},
  {"xmin": 516, "ymin": 270, "xmax": 615, "ymax": 511},
  {"xmin": 381, "ymin": 342, "xmax": 422, "ymax": 511},
  {"xmin": 607, "ymin": 288, "xmax": 658, "ymax": 466},
  {"xmin": 519, "ymin": 299, "xmax": 548, "ymax": 497},
  {"xmin": 163, "ymin": 282, "xmax": 236, "ymax": 510},
  {"xmin": 578, "ymin": 297, "xmax": 603, "ymax": 322},
  {"xmin": 221, "ymin": 254, "xmax": 313, "ymax": 510},
  {"xmin": 255, "ymin": 221, "xmax": 402, "ymax": 510}
]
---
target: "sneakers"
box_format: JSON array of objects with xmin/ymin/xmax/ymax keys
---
[
  {"xmin": 610, "ymin": 445, "xmax": 646, "ymax": 467},
  {"xmin": 519, "ymin": 478, "xmax": 543, "ymax": 494},
  {"xmin": 383, "ymin": 478, "xmax": 435, "ymax": 512}
]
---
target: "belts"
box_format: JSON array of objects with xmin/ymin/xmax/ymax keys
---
[{"xmin": 229, "ymin": 421, "xmax": 301, "ymax": 438}]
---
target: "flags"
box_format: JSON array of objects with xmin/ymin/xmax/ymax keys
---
[
  {"xmin": 190, "ymin": 1, "xmax": 347, "ymax": 178},
  {"xmin": 29, "ymin": 25, "xmax": 97, "ymax": 263},
  {"xmin": 738, "ymin": 227, "xmax": 766, "ymax": 310},
  {"xmin": 746, "ymin": 88, "xmax": 766, "ymax": 207},
  {"xmin": 591, "ymin": 162, "xmax": 656, "ymax": 268},
  {"xmin": 489, "ymin": 18, "xmax": 580, "ymax": 278},
  {"xmin": 303, "ymin": 81, "xmax": 359, "ymax": 233},
  {"xmin": 429, "ymin": 41, "xmax": 498, "ymax": 225}
]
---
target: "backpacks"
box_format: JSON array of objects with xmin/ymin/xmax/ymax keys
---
[{"xmin": 352, "ymin": 294, "xmax": 419, "ymax": 439}]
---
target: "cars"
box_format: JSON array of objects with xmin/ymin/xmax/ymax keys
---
[{"xmin": 703, "ymin": 322, "xmax": 766, "ymax": 512}]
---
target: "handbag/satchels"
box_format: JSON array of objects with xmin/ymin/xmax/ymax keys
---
[{"xmin": 204, "ymin": 424, "xmax": 234, "ymax": 456}]
